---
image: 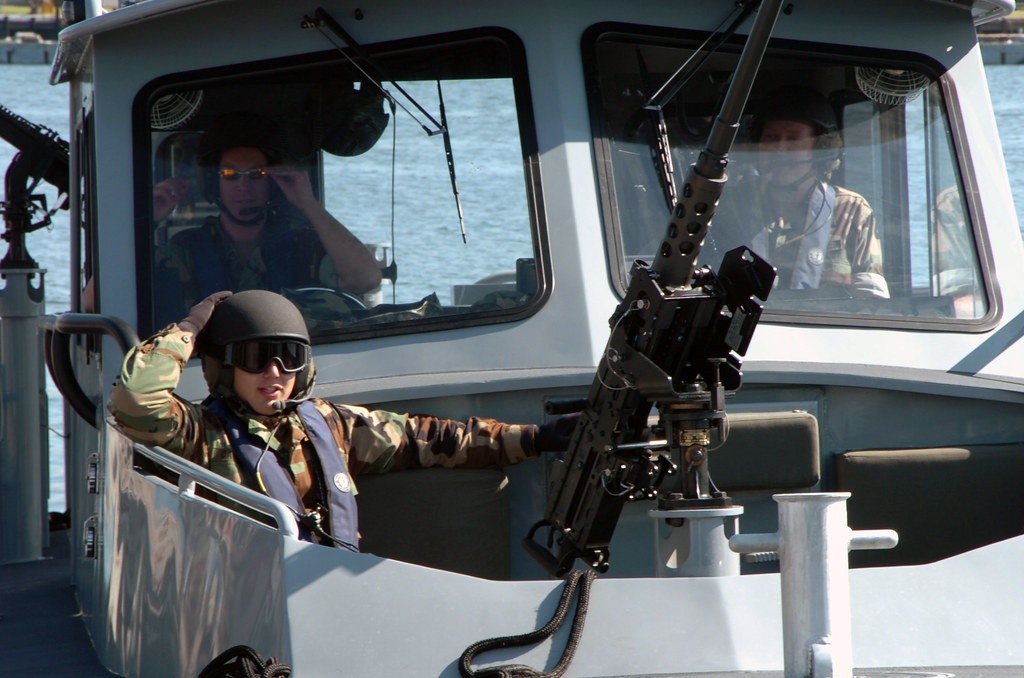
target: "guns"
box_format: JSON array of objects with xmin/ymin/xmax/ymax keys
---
[
  {"xmin": 0, "ymin": 104, "xmax": 88, "ymax": 210},
  {"xmin": 520, "ymin": 0, "xmax": 784, "ymax": 578}
]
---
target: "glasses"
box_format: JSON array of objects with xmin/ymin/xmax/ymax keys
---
[
  {"xmin": 217, "ymin": 168, "xmax": 268, "ymax": 182},
  {"xmin": 222, "ymin": 339, "xmax": 311, "ymax": 374}
]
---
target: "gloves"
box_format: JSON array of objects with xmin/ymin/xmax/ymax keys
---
[
  {"xmin": 535, "ymin": 412, "xmax": 580, "ymax": 451},
  {"xmin": 177, "ymin": 291, "xmax": 234, "ymax": 346}
]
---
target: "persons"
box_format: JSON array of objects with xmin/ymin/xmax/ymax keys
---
[
  {"xmin": 109, "ymin": 291, "xmax": 587, "ymax": 552},
  {"xmin": 73, "ymin": 125, "xmax": 387, "ymax": 327},
  {"xmin": 693, "ymin": 96, "xmax": 890, "ymax": 303}
]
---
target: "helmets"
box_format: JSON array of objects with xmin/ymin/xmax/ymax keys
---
[
  {"xmin": 196, "ymin": 111, "xmax": 284, "ymax": 204},
  {"xmin": 746, "ymin": 89, "xmax": 841, "ymax": 178},
  {"xmin": 196, "ymin": 290, "xmax": 311, "ymax": 400}
]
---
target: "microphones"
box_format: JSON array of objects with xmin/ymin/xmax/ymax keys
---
[
  {"xmin": 773, "ymin": 155, "xmax": 838, "ymax": 168},
  {"xmin": 272, "ymin": 376, "xmax": 316, "ymax": 411},
  {"xmin": 238, "ymin": 201, "xmax": 273, "ymax": 218}
]
